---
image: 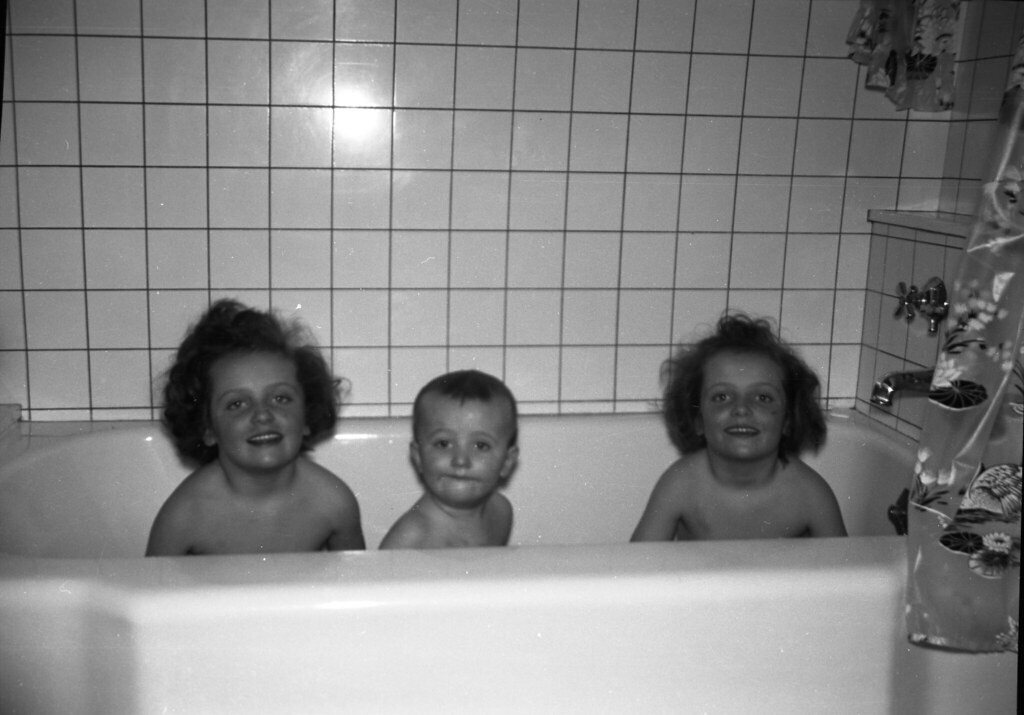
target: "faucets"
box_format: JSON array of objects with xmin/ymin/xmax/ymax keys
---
[
  {"xmin": 870, "ymin": 368, "xmax": 934, "ymax": 407},
  {"xmin": 891, "ymin": 277, "xmax": 949, "ymax": 332}
]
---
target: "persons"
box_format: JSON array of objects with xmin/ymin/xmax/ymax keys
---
[
  {"xmin": 630, "ymin": 315, "xmax": 848, "ymax": 541},
  {"xmin": 145, "ymin": 299, "xmax": 366, "ymax": 556},
  {"xmin": 377, "ymin": 370, "xmax": 519, "ymax": 549}
]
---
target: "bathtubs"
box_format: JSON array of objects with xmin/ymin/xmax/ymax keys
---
[{"xmin": 0, "ymin": 420, "xmax": 1017, "ymax": 715}]
